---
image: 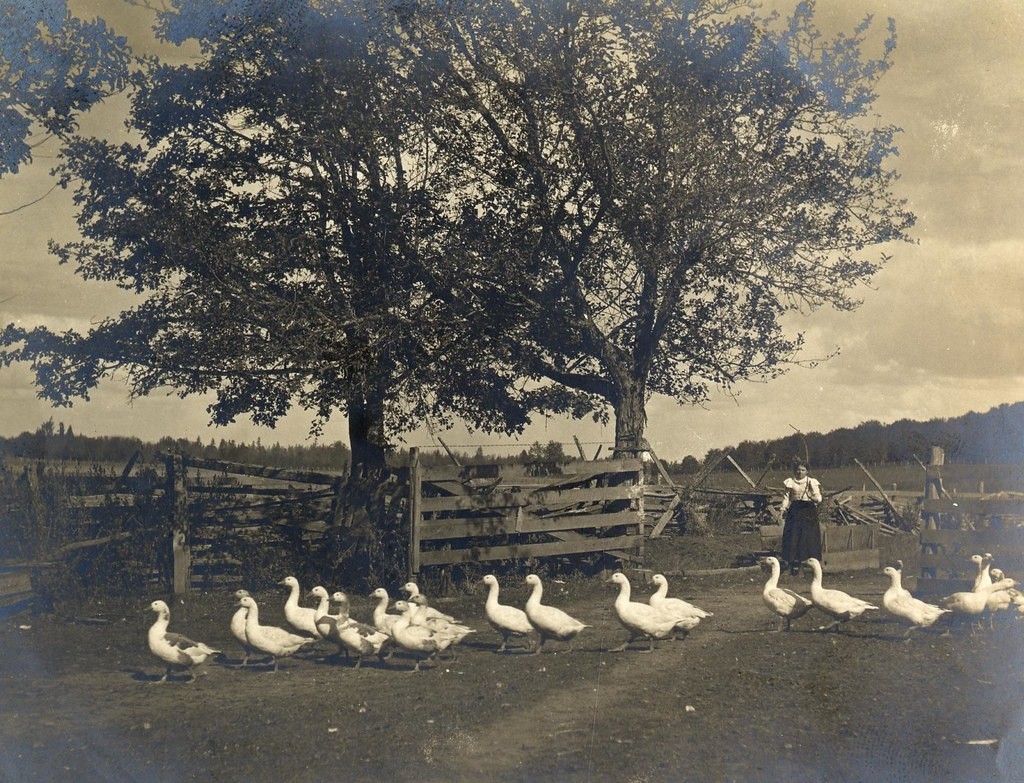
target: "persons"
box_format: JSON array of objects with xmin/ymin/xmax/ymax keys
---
[
  {"xmin": 778, "ymin": 461, "xmax": 822, "ymax": 578},
  {"xmin": 658, "ymin": 472, "xmax": 663, "ymax": 485}
]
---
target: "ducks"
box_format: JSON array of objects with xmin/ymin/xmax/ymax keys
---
[
  {"xmin": 800, "ymin": 557, "xmax": 879, "ymax": 632},
  {"xmin": 877, "ymin": 567, "xmax": 953, "ymax": 640},
  {"xmin": 605, "ymin": 572, "xmax": 715, "ymax": 653},
  {"xmin": 940, "ymin": 552, "xmax": 1024, "ymax": 633},
  {"xmin": 229, "ymin": 590, "xmax": 316, "ymax": 667},
  {"xmin": 143, "ymin": 600, "xmax": 221, "ymax": 685},
  {"xmin": 278, "ymin": 574, "xmax": 476, "ymax": 673},
  {"xmin": 760, "ymin": 556, "xmax": 812, "ymax": 633},
  {"xmin": 479, "ymin": 574, "xmax": 594, "ymax": 657}
]
